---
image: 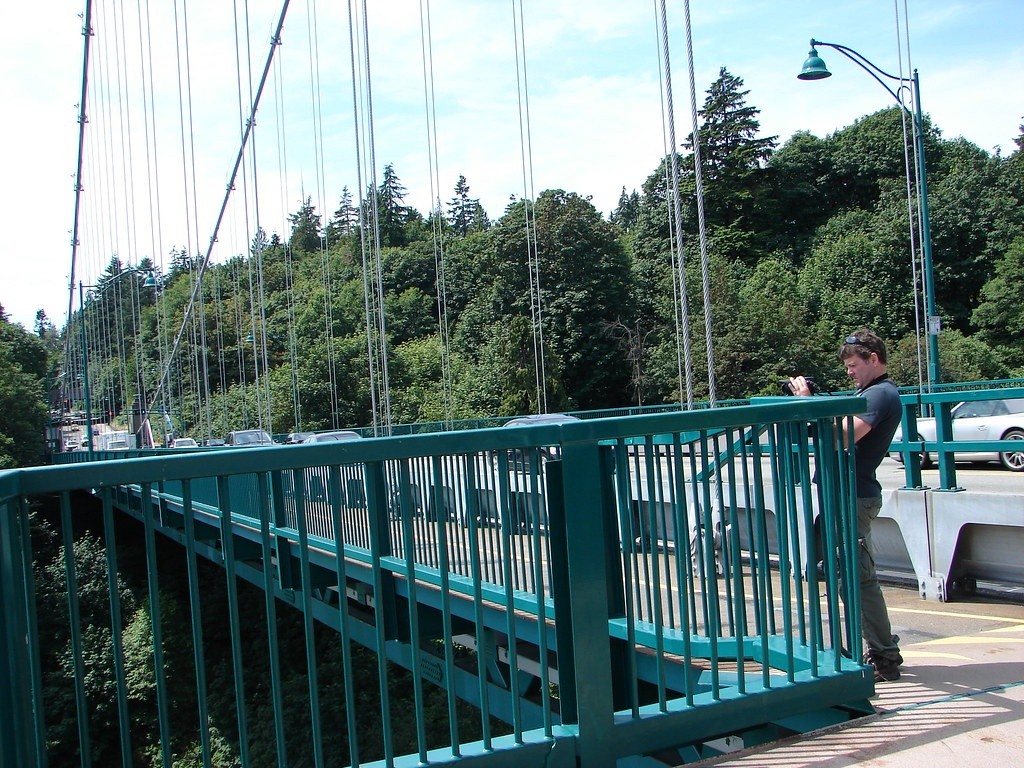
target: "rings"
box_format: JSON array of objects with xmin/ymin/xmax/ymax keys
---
[{"xmin": 796, "ymin": 388, "xmax": 800, "ymax": 390}]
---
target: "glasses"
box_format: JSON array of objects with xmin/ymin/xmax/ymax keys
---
[{"xmin": 843, "ymin": 336, "xmax": 880, "ymax": 353}]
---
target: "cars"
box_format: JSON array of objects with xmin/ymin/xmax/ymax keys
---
[
  {"xmin": 200, "ymin": 439, "xmax": 225, "ymax": 447},
  {"xmin": 222, "ymin": 429, "xmax": 282, "ymax": 446},
  {"xmin": 487, "ymin": 414, "xmax": 616, "ymax": 474},
  {"xmin": 64, "ymin": 441, "xmax": 79, "ymax": 451},
  {"xmin": 284, "ymin": 432, "xmax": 317, "ymax": 445},
  {"xmin": 888, "ymin": 398, "xmax": 1024, "ymax": 472},
  {"xmin": 169, "ymin": 438, "xmax": 201, "ymax": 448},
  {"xmin": 295, "ymin": 431, "xmax": 363, "ymax": 444},
  {"xmin": 63, "ymin": 434, "xmax": 76, "ymax": 441},
  {"xmin": 71, "ymin": 424, "xmax": 79, "ymax": 432},
  {"xmin": 80, "ymin": 435, "xmax": 88, "ymax": 440},
  {"xmin": 92, "ymin": 428, "xmax": 100, "ymax": 436},
  {"xmin": 102, "ymin": 440, "xmax": 130, "ymax": 450},
  {"xmin": 81, "ymin": 439, "xmax": 95, "ymax": 451}
]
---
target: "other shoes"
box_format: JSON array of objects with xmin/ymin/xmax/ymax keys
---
[{"xmin": 864, "ymin": 646, "xmax": 903, "ymax": 682}]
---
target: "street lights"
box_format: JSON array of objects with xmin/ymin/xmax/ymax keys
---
[
  {"xmin": 45, "ymin": 372, "xmax": 67, "ymax": 453},
  {"xmin": 798, "ymin": 38, "xmax": 941, "ymax": 392},
  {"xmin": 106, "ymin": 375, "xmax": 116, "ymax": 418},
  {"xmin": 80, "ymin": 266, "xmax": 162, "ymax": 454}
]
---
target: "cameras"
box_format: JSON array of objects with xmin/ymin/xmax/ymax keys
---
[{"xmin": 779, "ymin": 376, "xmax": 821, "ymax": 395}]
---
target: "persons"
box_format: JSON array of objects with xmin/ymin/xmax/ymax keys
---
[{"xmin": 788, "ymin": 331, "xmax": 903, "ymax": 683}]
